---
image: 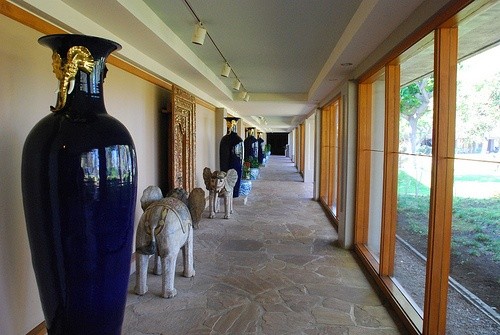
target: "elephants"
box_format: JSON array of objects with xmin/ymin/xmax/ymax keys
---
[
  {"xmin": 133, "ymin": 184, "xmax": 206, "ymax": 299},
  {"xmin": 202, "ymin": 166, "xmax": 238, "ymax": 220}
]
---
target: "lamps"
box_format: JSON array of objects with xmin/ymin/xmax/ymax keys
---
[
  {"xmin": 192, "ymin": 21, "xmax": 206, "ymax": 45},
  {"xmin": 240, "ymin": 91, "xmax": 250, "ymax": 102},
  {"xmin": 221, "ymin": 64, "xmax": 232, "ymax": 78},
  {"xmin": 233, "ymin": 80, "xmax": 241, "ymax": 90}
]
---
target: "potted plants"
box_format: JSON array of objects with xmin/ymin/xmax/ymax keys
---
[
  {"xmin": 245, "ymin": 156, "xmax": 260, "ymax": 180},
  {"xmin": 265, "ymin": 144, "xmax": 271, "ymax": 156}
]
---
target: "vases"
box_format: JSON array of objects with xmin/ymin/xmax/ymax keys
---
[{"xmin": 239, "ymin": 180, "xmax": 252, "ymax": 195}]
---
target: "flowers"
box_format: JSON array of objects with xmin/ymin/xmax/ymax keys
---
[{"xmin": 244, "ymin": 162, "xmax": 250, "ymax": 176}]
